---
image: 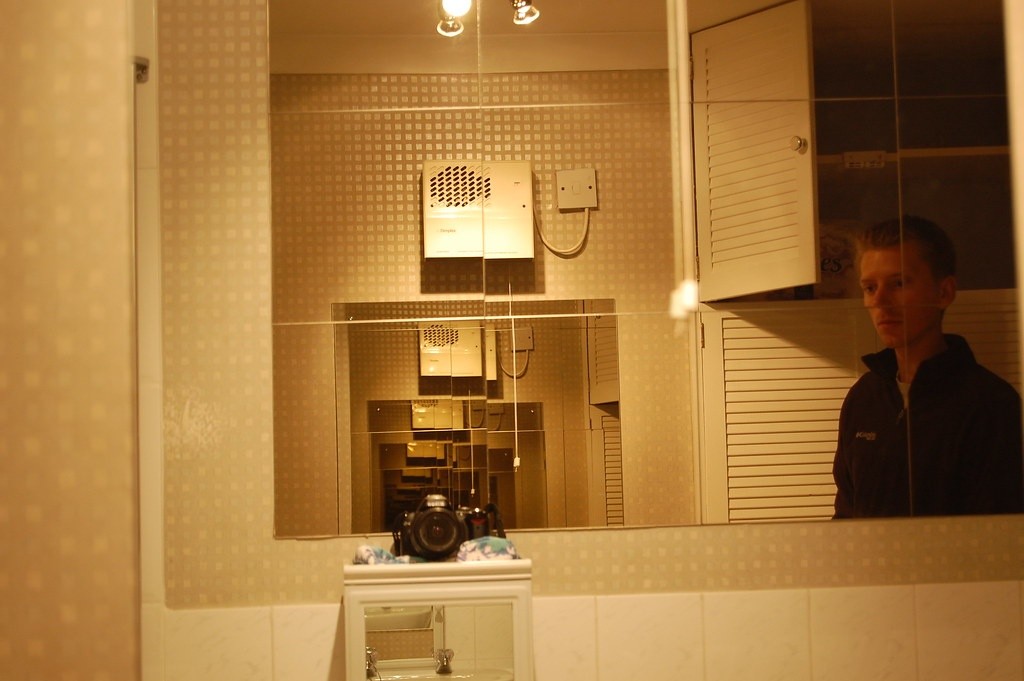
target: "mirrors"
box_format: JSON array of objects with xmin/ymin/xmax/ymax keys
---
[
  {"xmin": 361, "ymin": 600, "xmax": 515, "ymax": 681},
  {"xmin": 366, "ymin": 400, "xmax": 550, "ymax": 532},
  {"xmin": 263, "ymin": 0, "xmax": 1024, "ymax": 539},
  {"xmin": 379, "ymin": 445, "xmax": 492, "ymax": 522},
  {"xmin": 384, "ymin": 469, "xmax": 479, "ymax": 517},
  {"xmin": 327, "ymin": 298, "xmax": 628, "ymax": 529},
  {"xmin": 371, "ymin": 433, "xmax": 519, "ymax": 530}
]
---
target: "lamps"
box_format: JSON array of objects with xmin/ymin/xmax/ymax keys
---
[{"xmin": 436, "ymin": 0, "xmax": 541, "ymax": 38}]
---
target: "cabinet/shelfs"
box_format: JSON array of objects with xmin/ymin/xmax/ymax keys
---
[{"xmin": 689, "ymin": 0, "xmax": 1023, "ymax": 521}]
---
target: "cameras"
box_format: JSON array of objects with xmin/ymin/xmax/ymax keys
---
[{"xmin": 398, "ymin": 493, "xmax": 489, "ymax": 563}]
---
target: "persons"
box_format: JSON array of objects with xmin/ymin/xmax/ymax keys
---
[{"xmin": 831, "ymin": 213, "xmax": 1024, "ymax": 522}]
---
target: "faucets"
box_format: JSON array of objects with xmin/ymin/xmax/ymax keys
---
[
  {"xmin": 432, "ymin": 649, "xmax": 454, "ymax": 674},
  {"xmin": 366, "ymin": 646, "xmax": 378, "ymax": 676}
]
